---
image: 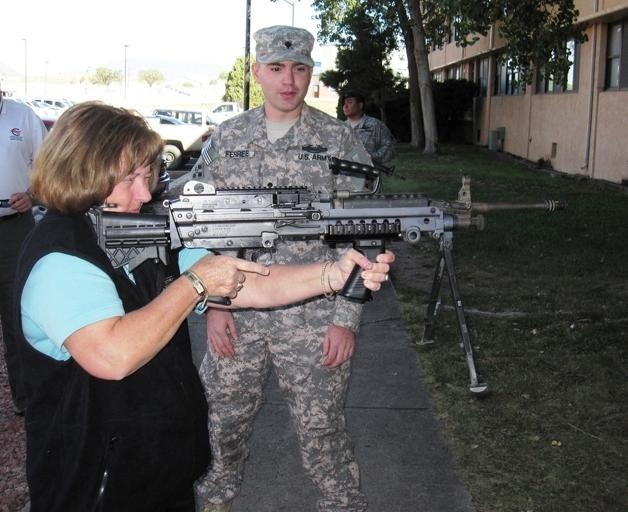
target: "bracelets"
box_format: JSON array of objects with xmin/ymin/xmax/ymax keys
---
[
  {"xmin": 321, "ymin": 261, "xmax": 333, "ymax": 298},
  {"xmin": 182, "ymin": 270, "xmax": 208, "ymax": 315}
]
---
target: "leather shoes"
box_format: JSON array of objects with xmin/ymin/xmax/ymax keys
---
[{"xmin": 11, "ymin": 400, "xmax": 27, "ymax": 415}]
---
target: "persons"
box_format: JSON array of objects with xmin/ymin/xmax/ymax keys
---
[
  {"xmin": 7, "ymin": 102, "xmax": 397, "ymax": 512},
  {"xmin": 340, "ymin": 90, "xmax": 397, "ymax": 166},
  {"xmin": 190, "ymin": 23, "xmax": 388, "ymax": 512},
  {"xmin": 0, "ymin": 82, "xmax": 53, "ymax": 418}
]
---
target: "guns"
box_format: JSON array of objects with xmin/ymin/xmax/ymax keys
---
[{"xmin": 90, "ymin": 156, "xmax": 568, "ymax": 393}]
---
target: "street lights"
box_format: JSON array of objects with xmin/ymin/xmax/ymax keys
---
[
  {"xmin": 271, "ymin": 0, "xmax": 304, "ymax": 28},
  {"xmin": 21, "ymin": 39, "xmax": 28, "ymax": 95},
  {"xmin": 120, "ymin": 42, "xmax": 131, "ymax": 88}
]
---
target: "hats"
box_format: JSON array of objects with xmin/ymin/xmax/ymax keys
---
[
  {"xmin": 342, "ymin": 90, "xmax": 363, "ymax": 99},
  {"xmin": 252, "ymin": 24, "xmax": 314, "ymax": 67}
]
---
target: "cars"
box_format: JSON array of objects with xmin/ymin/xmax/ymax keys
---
[{"xmin": 26, "ymin": 95, "xmax": 247, "ymax": 174}]
